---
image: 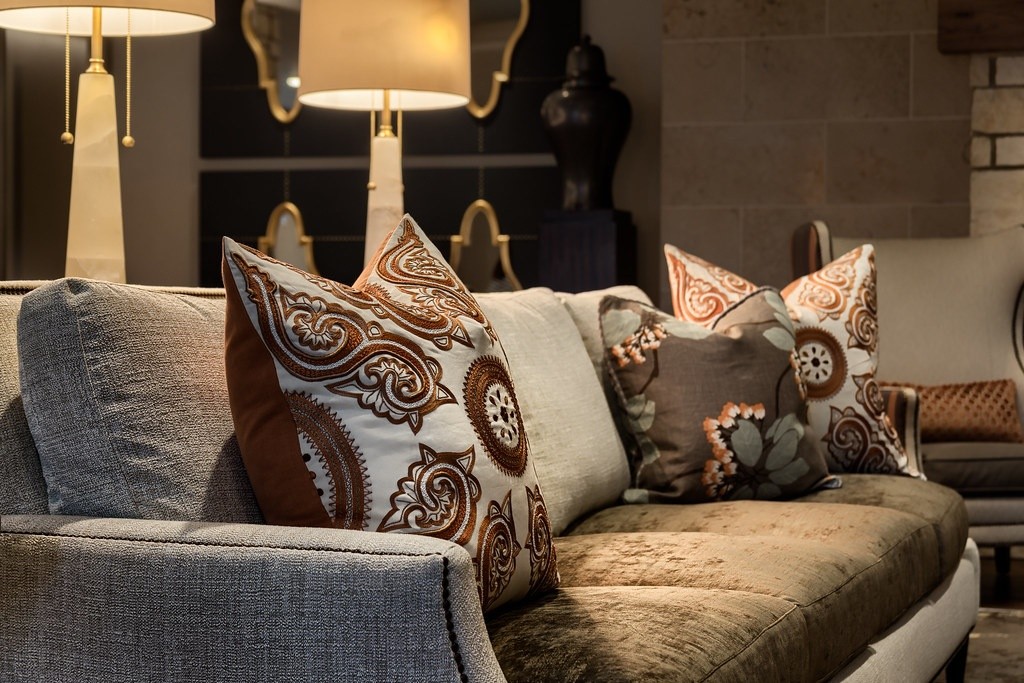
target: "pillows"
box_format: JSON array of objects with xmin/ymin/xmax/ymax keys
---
[
  {"xmin": 599, "ymin": 286, "xmax": 842, "ymax": 506},
  {"xmin": 222, "ymin": 214, "xmax": 561, "ymax": 615},
  {"xmin": 470, "ymin": 287, "xmax": 631, "ymax": 536},
  {"xmin": 879, "ymin": 380, "xmax": 1024, "ymax": 443},
  {"xmin": 563, "ymin": 285, "xmax": 654, "ymax": 389},
  {"xmin": 19, "ymin": 280, "xmax": 267, "ymax": 526},
  {"xmin": 664, "ymin": 243, "xmax": 929, "ymax": 481}
]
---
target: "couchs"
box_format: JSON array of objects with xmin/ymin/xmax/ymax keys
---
[{"xmin": 1, "ymin": 295, "xmax": 980, "ymax": 683}]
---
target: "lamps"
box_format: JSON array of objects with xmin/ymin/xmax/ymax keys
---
[
  {"xmin": 0, "ymin": 1, "xmax": 215, "ymax": 284},
  {"xmin": 242, "ymin": 0, "xmax": 529, "ymax": 268}
]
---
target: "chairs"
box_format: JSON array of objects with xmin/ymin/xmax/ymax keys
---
[{"xmin": 791, "ymin": 220, "xmax": 1024, "ymax": 573}]
